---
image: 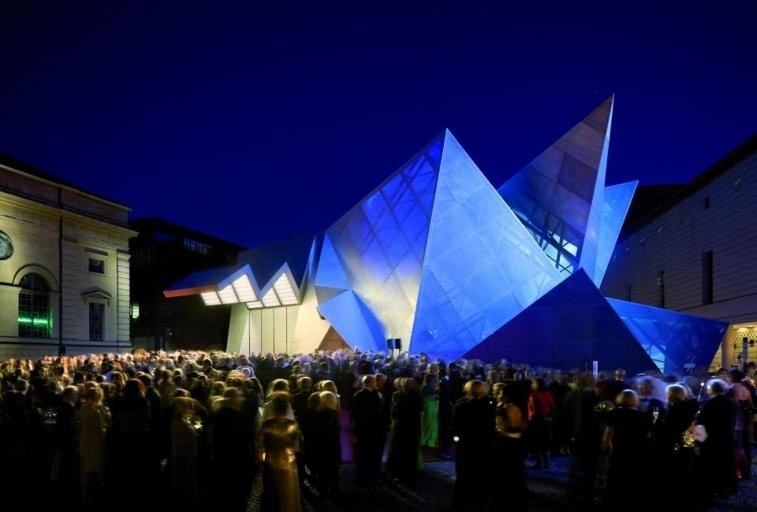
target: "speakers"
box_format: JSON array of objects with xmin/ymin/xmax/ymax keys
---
[{"xmin": 387, "ymin": 338, "xmax": 401, "ymax": 349}]
